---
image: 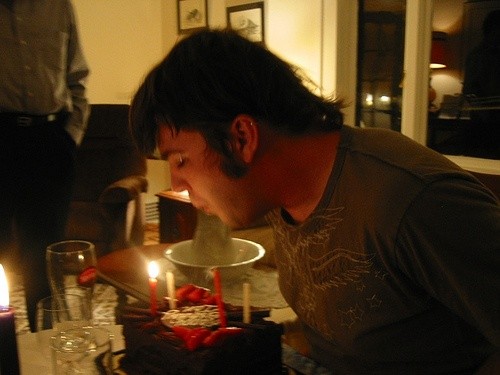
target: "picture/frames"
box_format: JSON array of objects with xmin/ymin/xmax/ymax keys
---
[
  {"xmin": 177, "ymin": 0, "xmax": 209, "ymax": 34},
  {"xmin": 226, "ymin": 1, "xmax": 265, "ymax": 45}
]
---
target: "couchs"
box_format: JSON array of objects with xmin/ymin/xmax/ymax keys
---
[{"xmin": 48, "ymin": 104, "xmax": 147, "ymax": 275}]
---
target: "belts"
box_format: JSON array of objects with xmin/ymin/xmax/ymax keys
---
[{"xmin": 2, "ymin": 108, "xmax": 69, "ymax": 128}]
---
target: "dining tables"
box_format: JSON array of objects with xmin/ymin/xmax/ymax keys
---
[{"xmin": 16, "ymin": 325, "xmax": 326, "ymax": 375}]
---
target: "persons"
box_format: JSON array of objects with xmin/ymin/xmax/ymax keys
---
[
  {"xmin": 128, "ymin": 29, "xmax": 500, "ymax": 375},
  {"xmin": 0, "ymin": 0, "xmax": 90, "ymax": 333}
]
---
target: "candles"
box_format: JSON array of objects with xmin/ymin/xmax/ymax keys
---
[
  {"xmin": 211, "ymin": 267, "xmax": 226, "ymax": 327},
  {"xmin": 242, "ymin": 283, "xmax": 251, "ymax": 324},
  {"xmin": 167, "ymin": 271, "xmax": 176, "ymax": 310},
  {"xmin": 0, "ymin": 263, "xmax": 20, "ymax": 375},
  {"xmin": 148, "ymin": 261, "xmax": 160, "ymax": 316}
]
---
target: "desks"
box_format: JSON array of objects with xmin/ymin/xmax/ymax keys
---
[{"xmin": 98, "ymin": 242, "xmax": 273, "ymax": 325}]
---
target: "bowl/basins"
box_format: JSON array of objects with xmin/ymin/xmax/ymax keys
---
[{"xmin": 162, "ymin": 237, "xmax": 266, "ymax": 288}]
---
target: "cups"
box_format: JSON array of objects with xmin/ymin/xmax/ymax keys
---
[
  {"xmin": 35, "ymin": 295, "xmax": 93, "ymax": 364},
  {"xmin": 45, "ymin": 241, "xmax": 95, "ymax": 320},
  {"xmin": 49, "ymin": 326, "xmax": 113, "ymax": 375}
]
